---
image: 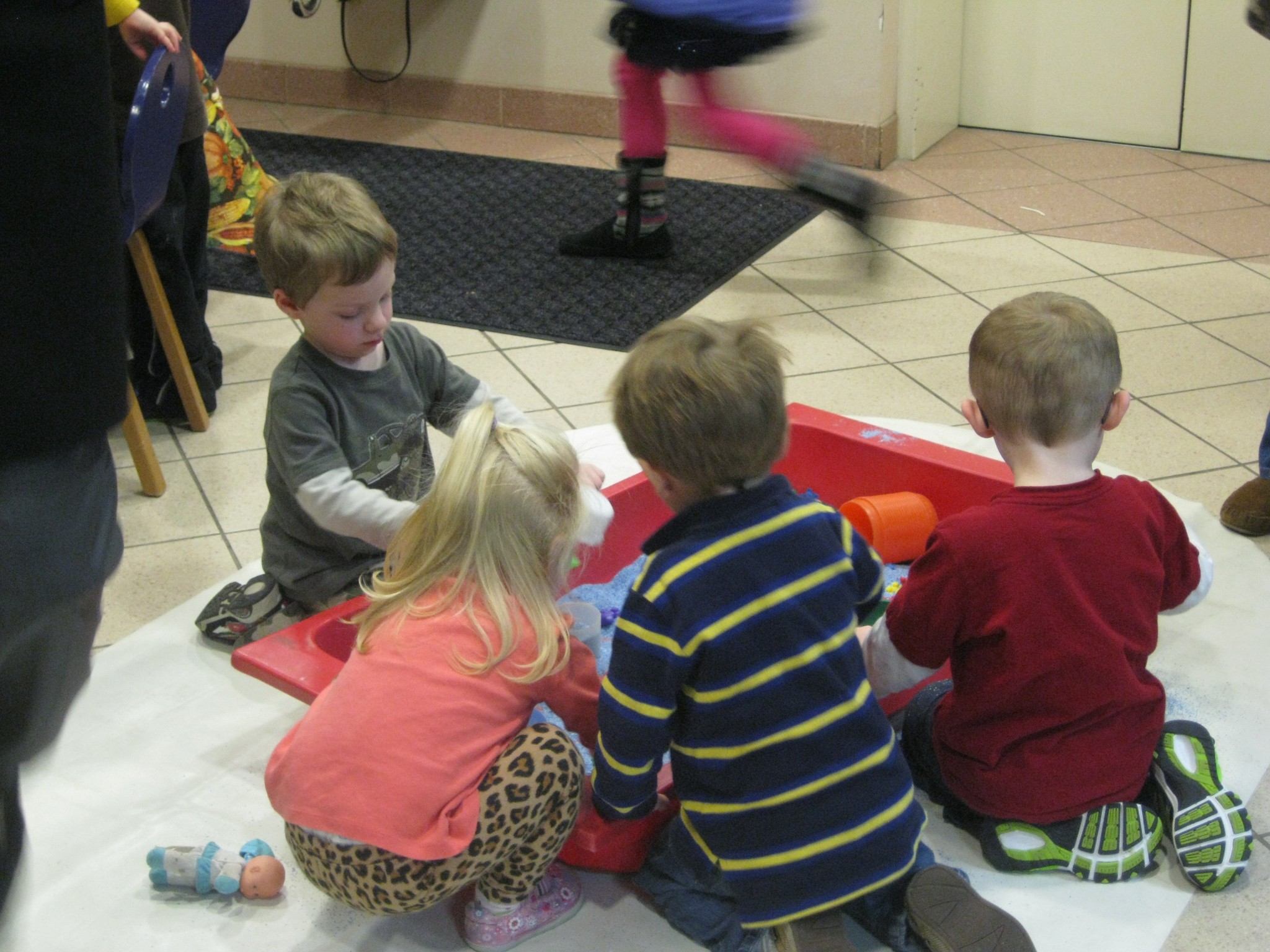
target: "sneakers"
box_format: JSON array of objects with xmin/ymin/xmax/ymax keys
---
[
  {"xmin": 464, "ymin": 862, "xmax": 584, "ymax": 950},
  {"xmin": 195, "ymin": 574, "xmax": 285, "ymax": 644},
  {"xmin": 1149, "ymin": 719, "xmax": 1254, "ymax": 891},
  {"xmin": 978, "ymin": 803, "xmax": 1163, "ymax": 882}
]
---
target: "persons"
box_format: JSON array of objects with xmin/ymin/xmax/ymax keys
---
[
  {"xmin": 0, "ymin": 0, "xmax": 250, "ymax": 909},
  {"xmin": 195, "ymin": 171, "xmax": 605, "ymax": 646},
  {"xmin": 264, "ymin": 403, "xmax": 602, "ymax": 952},
  {"xmin": 556, "ymin": 0, "xmax": 891, "ymax": 260},
  {"xmin": 147, "ymin": 839, "xmax": 285, "ymax": 900},
  {"xmin": 863, "ymin": 287, "xmax": 1254, "ymax": 895},
  {"xmin": 591, "ymin": 314, "xmax": 1037, "ymax": 952},
  {"xmin": 1219, "ymin": 0, "xmax": 1270, "ymax": 536}
]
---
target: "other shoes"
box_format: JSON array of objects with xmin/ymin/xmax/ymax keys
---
[
  {"xmin": 1220, "ymin": 477, "xmax": 1270, "ymax": 536},
  {"xmin": 775, "ymin": 912, "xmax": 892, "ymax": 952},
  {"xmin": 902, "ymin": 864, "xmax": 1037, "ymax": 951}
]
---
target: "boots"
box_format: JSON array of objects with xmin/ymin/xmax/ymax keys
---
[
  {"xmin": 559, "ymin": 148, "xmax": 674, "ymax": 259},
  {"xmin": 794, "ymin": 155, "xmax": 894, "ymax": 250}
]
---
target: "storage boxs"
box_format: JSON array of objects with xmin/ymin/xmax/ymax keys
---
[{"xmin": 234, "ymin": 404, "xmax": 1017, "ymax": 907}]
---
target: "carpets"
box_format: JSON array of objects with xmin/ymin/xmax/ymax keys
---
[
  {"xmin": 206, "ymin": 124, "xmax": 829, "ymax": 352},
  {"xmin": 0, "ymin": 418, "xmax": 1270, "ymax": 952}
]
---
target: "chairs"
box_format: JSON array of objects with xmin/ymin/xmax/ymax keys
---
[{"xmin": 121, "ymin": 39, "xmax": 207, "ymax": 497}]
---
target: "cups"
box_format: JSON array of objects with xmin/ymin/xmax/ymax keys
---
[{"xmin": 838, "ymin": 491, "xmax": 939, "ymax": 566}]
